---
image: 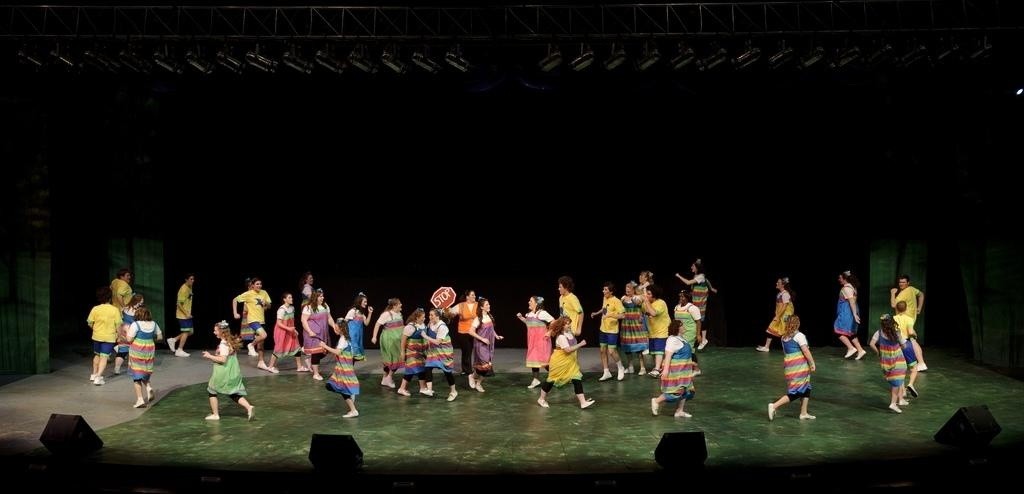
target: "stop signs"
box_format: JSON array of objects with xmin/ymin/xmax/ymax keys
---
[{"xmin": 430, "ymin": 286, "xmax": 456, "ymax": 309}]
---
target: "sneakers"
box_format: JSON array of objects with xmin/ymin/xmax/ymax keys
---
[
  {"xmin": 205, "ymin": 415, "xmax": 220, "ymax": 420},
  {"xmin": 651, "ymin": 398, "xmax": 660, "ymax": 416},
  {"xmin": 768, "ymin": 403, "xmax": 774, "ymax": 419},
  {"xmin": 844, "ymin": 347, "xmax": 867, "ymax": 360},
  {"xmin": 134, "ymin": 387, "xmax": 154, "ymax": 408},
  {"xmin": 697, "ymin": 340, "xmax": 708, "ymax": 350},
  {"xmin": 527, "ymin": 377, "xmax": 541, "ymax": 388},
  {"xmin": 599, "ymin": 365, "xmax": 660, "ymax": 381},
  {"xmin": 248, "ymin": 406, "xmax": 256, "ymax": 421},
  {"xmin": 581, "ymin": 398, "xmax": 595, "ymax": 409},
  {"xmin": 343, "ymin": 394, "xmax": 360, "ymax": 417},
  {"xmin": 757, "ymin": 346, "xmax": 768, "ymax": 351},
  {"xmin": 537, "ymin": 398, "xmax": 550, "ymax": 408},
  {"xmin": 247, "ymin": 343, "xmax": 324, "ymax": 381},
  {"xmin": 449, "ymin": 374, "xmax": 485, "ymax": 400},
  {"xmin": 799, "ymin": 414, "xmax": 816, "ymax": 419},
  {"xmin": 168, "ymin": 338, "xmax": 189, "ymax": 356},
  {"xmin": 114, "ymin": 365, "xmax": 121, "ymax": 375},
  {"xmin": 889, "ymin": 362, "xmax": 928, "ymax": 413},
  {"xmin": 90, "ymin": 373, "xmax": 105, "ymax": 385},
  {"xmin": 674, "ymin": 413, "xmax": 692, "ymax": 418},
  {"xmin": 381, "ymin": 374, "xmax": 435, "ymax": 396}
]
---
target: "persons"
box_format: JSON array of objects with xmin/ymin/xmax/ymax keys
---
[
  {"xmin": 768, "ymin": 314, "xmax": 816, "ymax": 421},
  {"xmin": 755, "ymin": 278, "xmax": 796, "ymax": 352},
  {"xmin": 234, "ymin": 274, "xmax": 374, "ymax": 418},
  {"xmin": 88, "ymin": 270, "xmax": 162, "ymax": 407},
  {"xmin": 517, "ymin": 259, "xmax": 719, "ymax": 418},
  {"xmin": 892, "ymin": 301, "xmax": 919, "ymax": 398},
  {"xmin": 372, "ymin": 289, "xmax": 503, "ymax": 401},
  {"xmin": 201, "ymin": 319, "xmax": 255, "ymax": 422},
  {"xmin": 833, "ymin": 271, "xmax": 867, "ymax": 361},
  {"xmin": 167, "ymin": 274, "xmax": 194, "ymax": 357},
  {"xmin": 891, "ymin": 276, "xmax": 929, "ymax": 372},
  {"xmin": 869, "ymin": 314, "xmax": 910, "ymax": 414}
]
